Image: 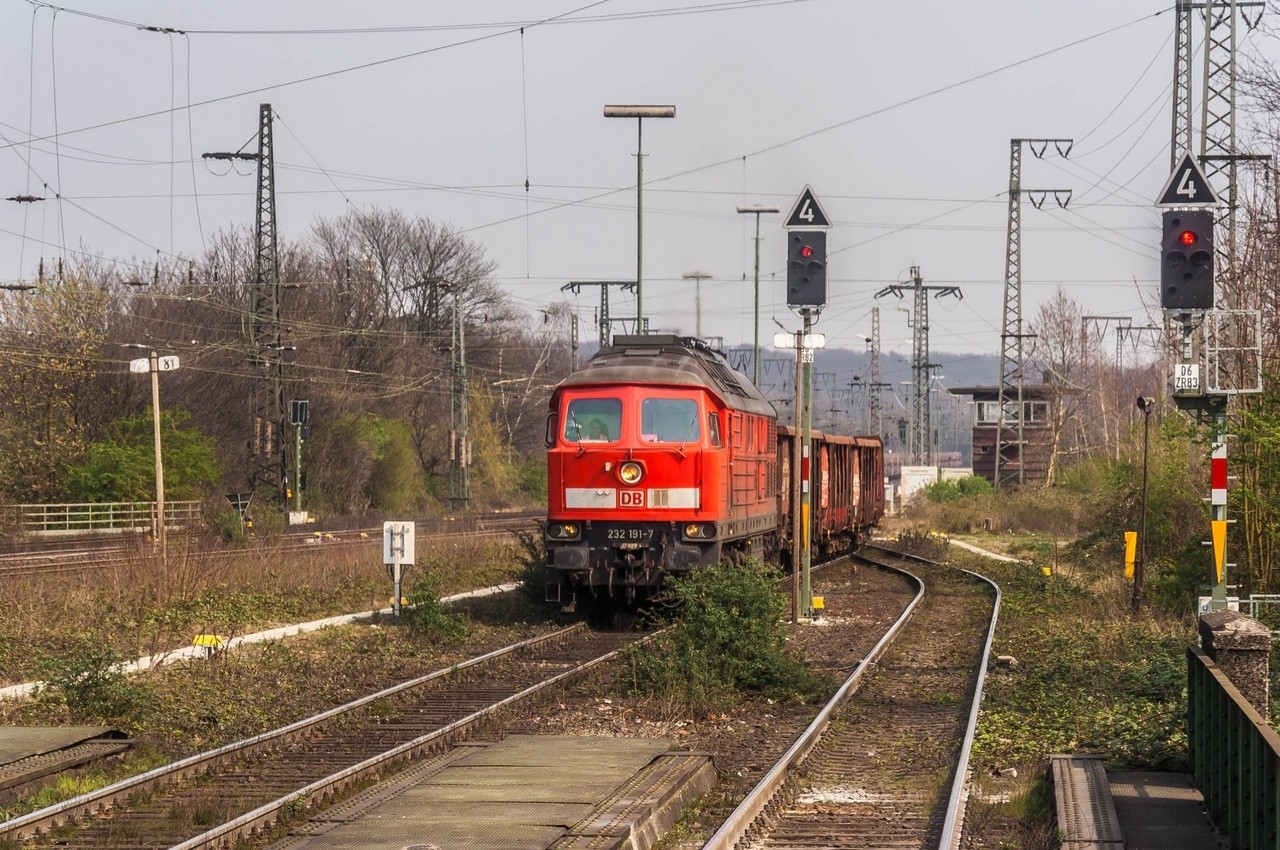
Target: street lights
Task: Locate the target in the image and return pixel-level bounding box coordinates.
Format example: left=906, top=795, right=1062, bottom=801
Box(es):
left=773, top=333, right=826, bottom=624
left=682, top=272, right=713, bottom=337
left=737, top=205, right=780, bottom=389
left=602, top=102, right=677, bottom=335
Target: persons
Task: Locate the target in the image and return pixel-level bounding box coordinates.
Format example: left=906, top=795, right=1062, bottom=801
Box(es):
left=582, top=419, right=608, bottom=440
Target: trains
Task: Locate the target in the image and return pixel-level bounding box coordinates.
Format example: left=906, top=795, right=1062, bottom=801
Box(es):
left=540, top=333, right=886, bottom=621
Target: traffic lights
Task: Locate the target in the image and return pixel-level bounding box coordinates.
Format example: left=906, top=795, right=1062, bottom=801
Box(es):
left=788, top=231, right=827, bottom=305
left=1161, top=211, right=1214, bottom=309
